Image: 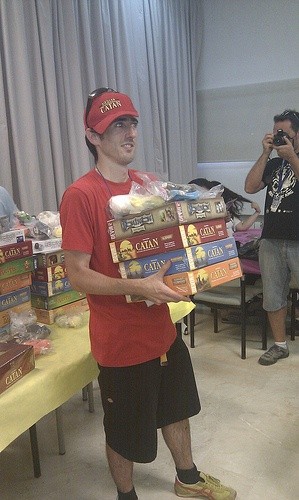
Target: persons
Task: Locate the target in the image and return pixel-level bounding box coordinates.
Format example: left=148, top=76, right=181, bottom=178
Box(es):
left=0, top=186, right=19, bottom=225
left=59, top=88, right=238, bottom=500
left=187, top=178, right=261, bottom=238
left=244, top=109, right=299, bottom=366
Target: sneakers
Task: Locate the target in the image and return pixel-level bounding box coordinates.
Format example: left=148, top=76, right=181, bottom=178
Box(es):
left=258, top=344, right=289, bottom=366
left=174, top=471, right=237, bottom=500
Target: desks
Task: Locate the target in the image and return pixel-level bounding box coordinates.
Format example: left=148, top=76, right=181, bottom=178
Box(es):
left=234, top=230, right=299, bottom=342
left=0, top=301, right=197, bottom=483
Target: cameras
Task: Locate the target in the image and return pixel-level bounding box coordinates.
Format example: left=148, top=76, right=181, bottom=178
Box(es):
left=272, top=130, right=290, bottom=146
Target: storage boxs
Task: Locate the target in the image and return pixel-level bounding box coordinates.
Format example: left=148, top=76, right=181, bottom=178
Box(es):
left=0, top=195, right=244, bottom=396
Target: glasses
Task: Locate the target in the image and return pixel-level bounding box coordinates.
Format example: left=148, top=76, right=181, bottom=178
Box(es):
left=281, top=110, right=299, bottom=123
left=86, top=88, right=119, bottom=128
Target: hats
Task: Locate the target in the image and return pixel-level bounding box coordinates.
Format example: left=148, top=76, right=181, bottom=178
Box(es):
left=84, top=91, right=139, bottom=134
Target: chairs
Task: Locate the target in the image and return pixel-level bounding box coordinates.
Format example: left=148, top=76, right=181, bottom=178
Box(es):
left=188, top=272, right=269, bottom=360
